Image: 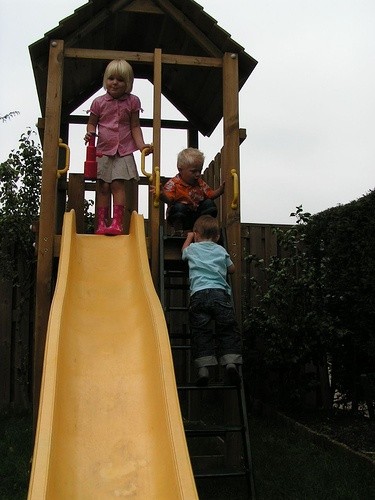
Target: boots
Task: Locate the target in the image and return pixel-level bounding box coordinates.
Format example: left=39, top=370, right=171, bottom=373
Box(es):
left=106, top=204, right=126, bottom=234
left=94, top=207, right=110, bottom=235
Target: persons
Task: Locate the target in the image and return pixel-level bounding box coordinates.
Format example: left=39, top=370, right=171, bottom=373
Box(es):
left=83, top=60, right=153, bottom=235
left=182, top=215, right=243, bottom=387
left=158, top=148, right=226, bottom=236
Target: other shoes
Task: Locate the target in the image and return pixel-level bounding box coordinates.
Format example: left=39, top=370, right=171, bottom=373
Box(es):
left=224, top=364, right=241, bottom=385
left=197, top=368, right=211, bottom=387
